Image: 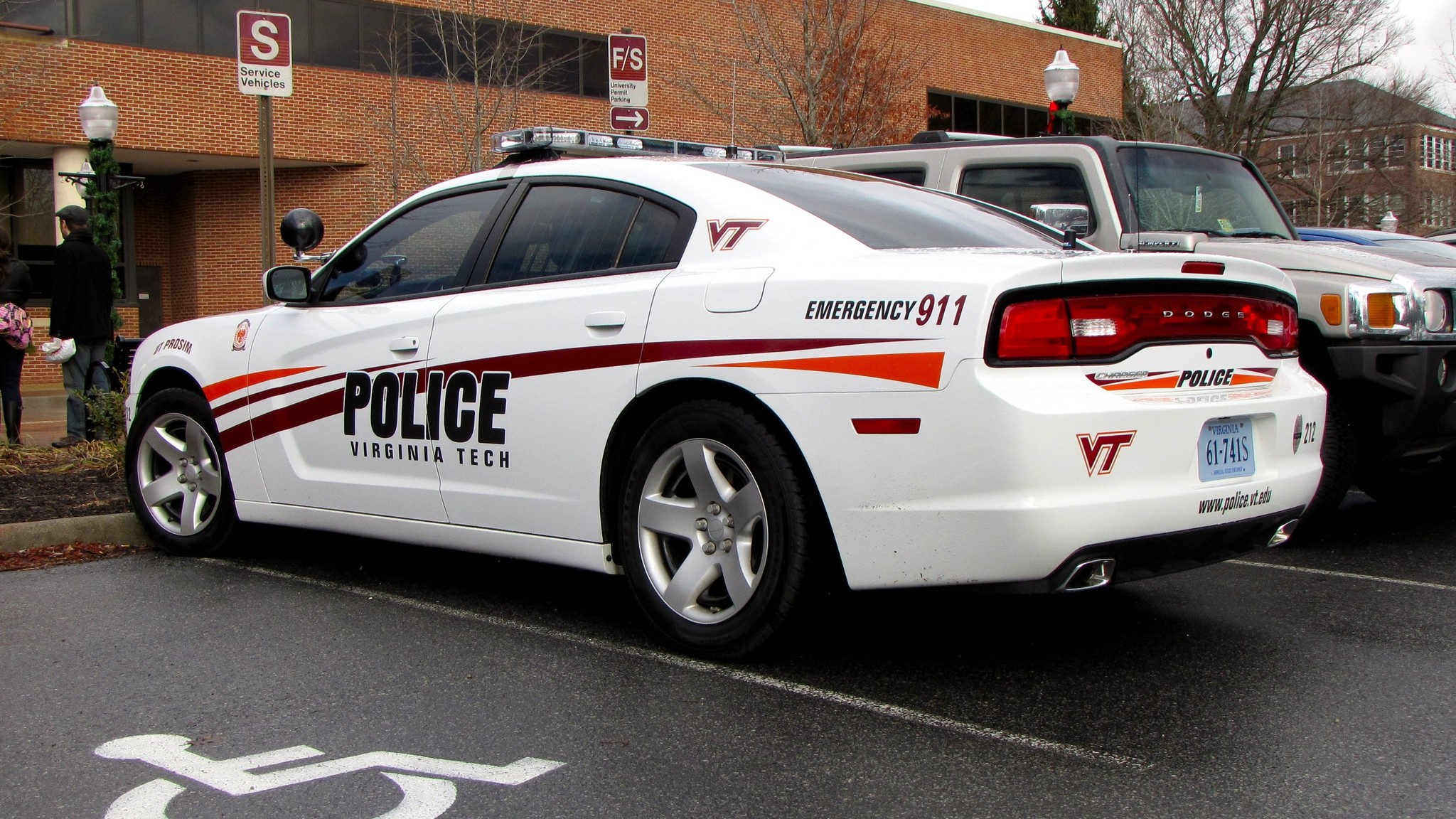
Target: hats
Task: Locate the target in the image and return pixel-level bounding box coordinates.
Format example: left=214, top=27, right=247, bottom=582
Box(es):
left=55, top=205, right=88, bottom=224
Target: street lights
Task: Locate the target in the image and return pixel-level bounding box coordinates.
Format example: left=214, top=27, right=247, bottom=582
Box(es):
left=58, top=86, right=145, bottom=391
left=1044, top=43, right=1080, bottom=137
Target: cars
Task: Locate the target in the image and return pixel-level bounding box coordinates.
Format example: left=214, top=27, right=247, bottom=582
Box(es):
left=1212, top=226, right=1456, bottom=261
left=122, top=126, right=1331, bottom=662
left=1419, top=228, right=1455, bottom=245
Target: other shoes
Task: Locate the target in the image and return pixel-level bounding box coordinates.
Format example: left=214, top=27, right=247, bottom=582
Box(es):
left=10, top=435, right=111, bottom=449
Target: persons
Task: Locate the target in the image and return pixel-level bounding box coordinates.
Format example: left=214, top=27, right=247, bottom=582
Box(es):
left=49, top=205, right=113, bottom=448
left=0, top=227, right=33, bottom=448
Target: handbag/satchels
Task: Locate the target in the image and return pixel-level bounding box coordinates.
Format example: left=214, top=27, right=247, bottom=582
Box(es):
left=0, top=302, right=34, bottom=349
left=42, top=337, right=77, bottom=365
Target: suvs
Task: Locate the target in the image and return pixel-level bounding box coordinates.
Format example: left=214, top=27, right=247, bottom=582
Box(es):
left=773, top=131, right=1456, bottom=549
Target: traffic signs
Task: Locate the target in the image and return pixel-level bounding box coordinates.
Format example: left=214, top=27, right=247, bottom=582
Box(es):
left=608, top=34, right=649, bottom=132
